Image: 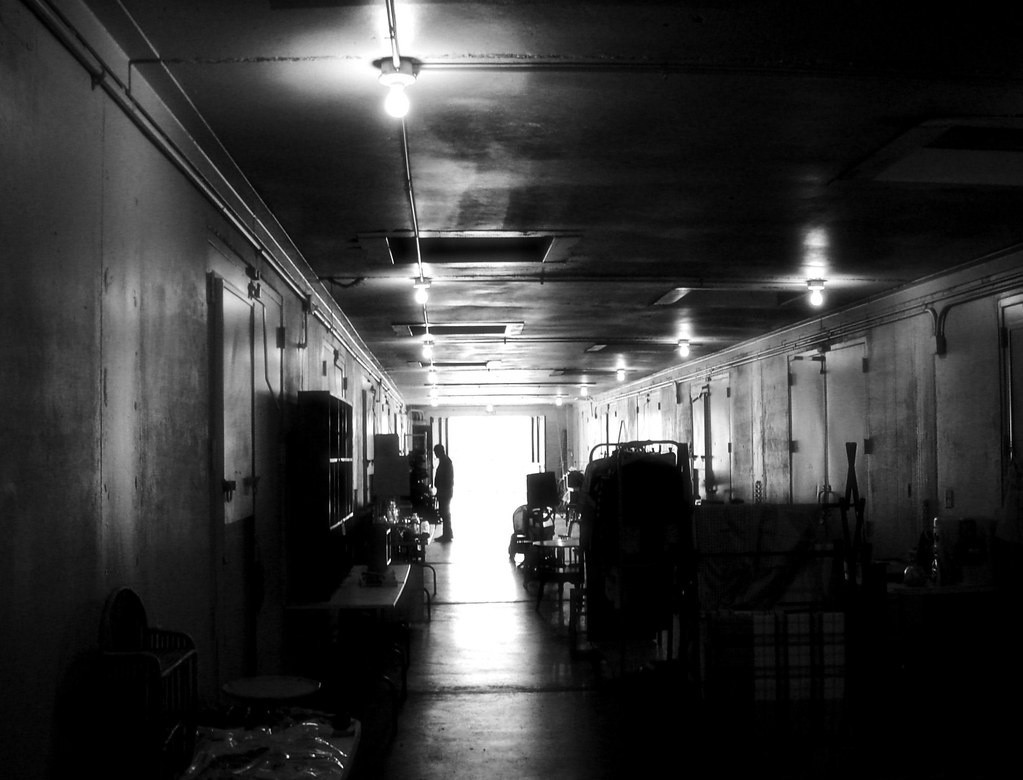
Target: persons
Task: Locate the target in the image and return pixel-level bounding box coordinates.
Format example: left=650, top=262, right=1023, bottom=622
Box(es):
left=433, top=445, right=452, bottom=543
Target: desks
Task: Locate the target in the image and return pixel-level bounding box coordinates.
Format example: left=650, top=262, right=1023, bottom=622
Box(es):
left=325, top=563, right=412, bottom=627
left=181, top=713, right=362, bottom=779
left=533, top=538, right=583, bottom=606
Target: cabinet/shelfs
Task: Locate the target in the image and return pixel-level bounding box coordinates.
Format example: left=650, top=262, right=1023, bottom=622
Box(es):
left=296, top=392, right=355, bottom=529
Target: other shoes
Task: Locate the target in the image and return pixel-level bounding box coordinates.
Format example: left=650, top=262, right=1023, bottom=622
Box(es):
left=435, top=536, right=450, bottom=543
